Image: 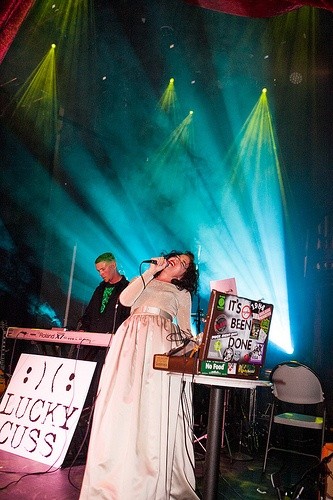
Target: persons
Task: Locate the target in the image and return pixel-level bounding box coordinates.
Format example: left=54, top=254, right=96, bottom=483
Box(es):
left=79, top=250, right=200, bottom=500
left=76, top=252, right=131, bottom=395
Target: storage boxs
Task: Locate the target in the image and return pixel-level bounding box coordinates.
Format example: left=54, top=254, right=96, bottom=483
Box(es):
left=152, top=289, right=274, bottom=380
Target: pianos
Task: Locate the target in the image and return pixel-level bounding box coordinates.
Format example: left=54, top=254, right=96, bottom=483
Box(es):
left=6, top=326, right=116, bottom=456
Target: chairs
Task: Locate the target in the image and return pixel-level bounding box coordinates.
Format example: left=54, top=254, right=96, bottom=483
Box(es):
left=263, top=361, right=328, bottom=490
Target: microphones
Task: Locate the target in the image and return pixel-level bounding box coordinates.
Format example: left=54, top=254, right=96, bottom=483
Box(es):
left=142, top=259, right=170, bottom=268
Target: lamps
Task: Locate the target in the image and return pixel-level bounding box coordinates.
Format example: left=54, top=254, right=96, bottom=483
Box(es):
left=289, top=68, right=304, bottom=84
left=159, top=22, right=179, bottom=38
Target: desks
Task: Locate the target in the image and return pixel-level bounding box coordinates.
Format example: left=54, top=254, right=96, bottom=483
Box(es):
left=168, top=372, right=273, bottom=500
